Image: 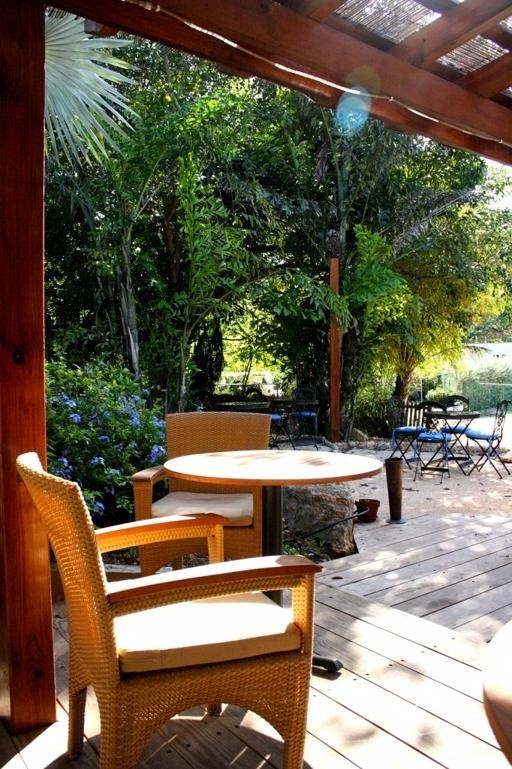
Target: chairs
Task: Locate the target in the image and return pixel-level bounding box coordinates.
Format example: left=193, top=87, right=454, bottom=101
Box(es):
left=129, top=411, right=273, bottom=578
left=387, top=395, right=508, bottom=480
left=15, top=451, right=316, bottom=768
left=243, top=389, right=323, bottom=452
